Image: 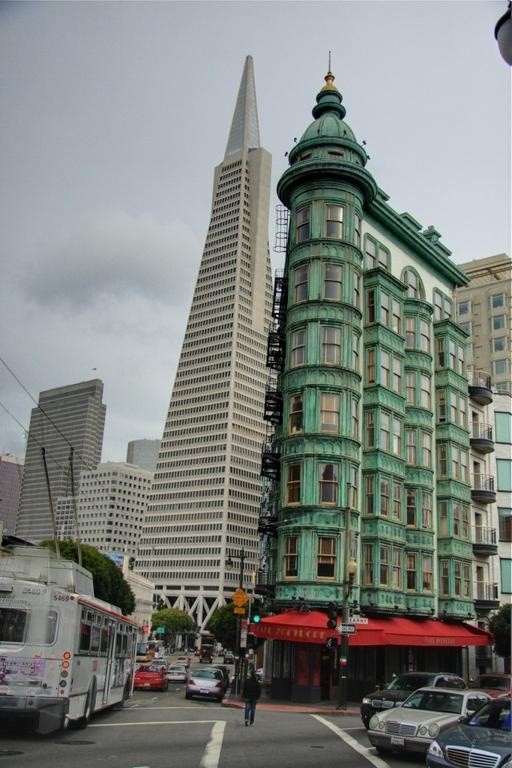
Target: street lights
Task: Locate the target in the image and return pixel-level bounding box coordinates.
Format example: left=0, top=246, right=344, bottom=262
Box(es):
left=337, top=559, right=359, bottom=709
left=225, top=548, right=248, bottom=658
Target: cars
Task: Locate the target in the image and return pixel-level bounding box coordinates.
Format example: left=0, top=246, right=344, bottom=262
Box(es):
left=360, top=673, right=512, bottom=768
left=199, top=644, right=214, bottom=664
left=134, top=654, right=234, bottom=703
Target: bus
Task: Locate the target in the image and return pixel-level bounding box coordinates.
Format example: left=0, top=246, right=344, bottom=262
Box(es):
left=0, top=546, right=140, bottom=736
left=136, top=640, right=165, bottom=662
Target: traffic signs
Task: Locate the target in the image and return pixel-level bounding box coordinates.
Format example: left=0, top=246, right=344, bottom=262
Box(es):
left=337, top=624, right=355, bottom=635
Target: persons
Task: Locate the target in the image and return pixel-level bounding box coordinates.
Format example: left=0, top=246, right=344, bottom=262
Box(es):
left=240, top=669, right=261, bottom=727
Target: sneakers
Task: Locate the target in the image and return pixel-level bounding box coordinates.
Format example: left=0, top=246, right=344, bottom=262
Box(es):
left=244, top=720, right=254, bottom=726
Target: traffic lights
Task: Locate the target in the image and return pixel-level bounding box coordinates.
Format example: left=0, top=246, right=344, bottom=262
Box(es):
left=251, top=598, right=262, bottom=624
left=326, top=600, right=339, bottom=629
left=326, top=637, right=337, bottom=648
